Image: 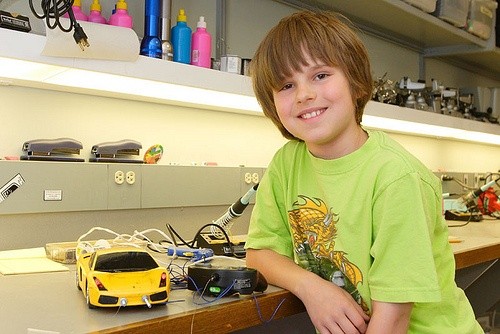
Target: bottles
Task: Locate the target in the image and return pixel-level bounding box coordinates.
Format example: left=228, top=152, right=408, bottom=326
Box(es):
left=88, top=0, right=107, bottom=24
left=171, top=9, right=192, bottom=64
left=64, top=0, right=88, bottom=20
left=192, top=17, right=211, bottom=69
left=109, top=0, right=132, bottom=29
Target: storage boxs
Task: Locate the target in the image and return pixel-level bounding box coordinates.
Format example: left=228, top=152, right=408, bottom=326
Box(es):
left=403, top=0, right=497, bottom=40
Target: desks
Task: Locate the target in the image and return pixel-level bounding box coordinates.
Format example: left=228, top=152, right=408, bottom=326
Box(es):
left=0, top=214, right=500, bottom=334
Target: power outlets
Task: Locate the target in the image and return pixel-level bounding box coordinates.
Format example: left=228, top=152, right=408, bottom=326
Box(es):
left=479, top=175, right=484, bottom=180
left=245, top=173, right=251, bottom=184
left=441, top=175, right=447, bottom=181
left=114, top=171, right=124, bottom=184
left=252, top=173, right=258, bottom=184
left=126, top=171, right=136, bottom=184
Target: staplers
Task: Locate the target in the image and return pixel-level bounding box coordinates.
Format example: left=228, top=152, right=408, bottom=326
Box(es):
left=88, top=137, right=145, bottom=164
left=20, top=136, right=86, bottom=163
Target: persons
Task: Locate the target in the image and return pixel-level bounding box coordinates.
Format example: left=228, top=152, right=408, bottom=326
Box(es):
left=244, top=11, right=486, bottom=334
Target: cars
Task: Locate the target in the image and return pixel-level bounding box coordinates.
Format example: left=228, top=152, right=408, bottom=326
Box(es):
left=76, top=243, right=171, bottom=309
